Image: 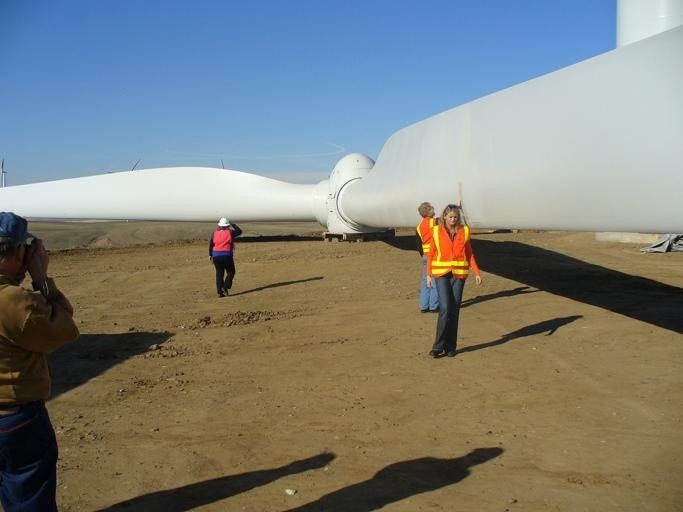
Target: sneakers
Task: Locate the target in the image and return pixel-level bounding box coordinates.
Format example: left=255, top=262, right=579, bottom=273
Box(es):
left=218, top=287, right=228, bottom=297
left=421, top=306, right=439, bottom=313
left=429, top=344, right=457, bottom=357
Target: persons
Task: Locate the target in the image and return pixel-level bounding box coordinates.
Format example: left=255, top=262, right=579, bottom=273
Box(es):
left=415, top=198, right=461, bottom=313
left=208, top=217, right=242, bottom=298
left=0, top=211, right=79, bottom=511
left=426, top=204, right=482, bottom=357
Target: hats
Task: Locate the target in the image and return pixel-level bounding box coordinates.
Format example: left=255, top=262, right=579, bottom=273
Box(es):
left=217, top=217, right=231, bottom=227
left=0, top=212, right=34, bottom=238
